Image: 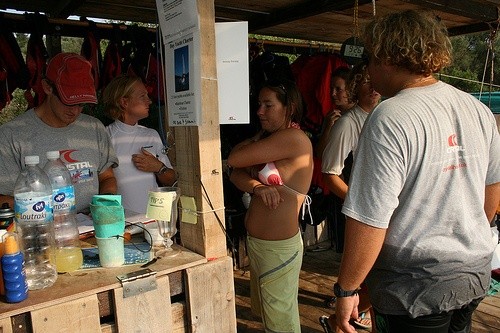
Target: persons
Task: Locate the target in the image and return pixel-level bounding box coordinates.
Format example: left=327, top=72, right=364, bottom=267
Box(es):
left=314, top=8, right=500, bottom=333
left=104, top=74, right=176, bottom=214
left=0, top=52, right=119, bottom=215
left=222, top=80, right=314, bottom=333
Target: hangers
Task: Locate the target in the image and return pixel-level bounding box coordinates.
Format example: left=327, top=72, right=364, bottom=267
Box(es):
left=0, top=11, right=164, bottom=46
left=249, top=38, right=348, bottom=67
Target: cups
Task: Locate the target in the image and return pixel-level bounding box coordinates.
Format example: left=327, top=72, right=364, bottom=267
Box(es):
left=95, top=233, right=124, bottom=269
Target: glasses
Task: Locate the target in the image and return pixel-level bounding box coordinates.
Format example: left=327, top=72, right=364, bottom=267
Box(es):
left=276, top=78, right=288, bottom=93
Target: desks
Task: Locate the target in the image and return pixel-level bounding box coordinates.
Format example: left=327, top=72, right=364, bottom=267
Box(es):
left=0, top=236, right=207, bottom=333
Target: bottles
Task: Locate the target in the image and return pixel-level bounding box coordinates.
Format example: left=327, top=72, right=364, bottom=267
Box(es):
left=43, top=151, right=83, bottom=274
left=13, top=155, right=58, bottom=291
left=1, top=237, right=29, bottom=303
left=0, top=202, right=15, bottom=232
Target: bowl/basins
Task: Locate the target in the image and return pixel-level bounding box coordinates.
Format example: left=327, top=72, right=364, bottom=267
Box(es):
left=143, top=221, right=177, bottom=246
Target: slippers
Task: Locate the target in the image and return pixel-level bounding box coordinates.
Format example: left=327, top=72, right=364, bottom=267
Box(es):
left=354, top=312, right=372, bottom=328
left=319, top=314, right=333, bottom=333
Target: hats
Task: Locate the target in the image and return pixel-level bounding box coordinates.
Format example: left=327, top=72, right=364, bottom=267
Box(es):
left=46, top=52, right=98, bottom=106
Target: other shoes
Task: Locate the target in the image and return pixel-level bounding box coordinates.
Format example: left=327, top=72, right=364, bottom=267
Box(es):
left=327, top=296, right=337, bottom=308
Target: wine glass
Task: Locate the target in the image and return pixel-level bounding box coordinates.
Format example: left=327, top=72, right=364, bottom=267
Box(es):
left=150, top=186, right=181, bottom=260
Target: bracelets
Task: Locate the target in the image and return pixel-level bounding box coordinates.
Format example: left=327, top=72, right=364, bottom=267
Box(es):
left=253, top=184, right=263, bottom=192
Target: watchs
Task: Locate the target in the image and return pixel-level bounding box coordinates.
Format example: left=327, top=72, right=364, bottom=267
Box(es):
left=334, top=283, right=355, bottom=298
left=153, top=163, right=166, bottom=174
